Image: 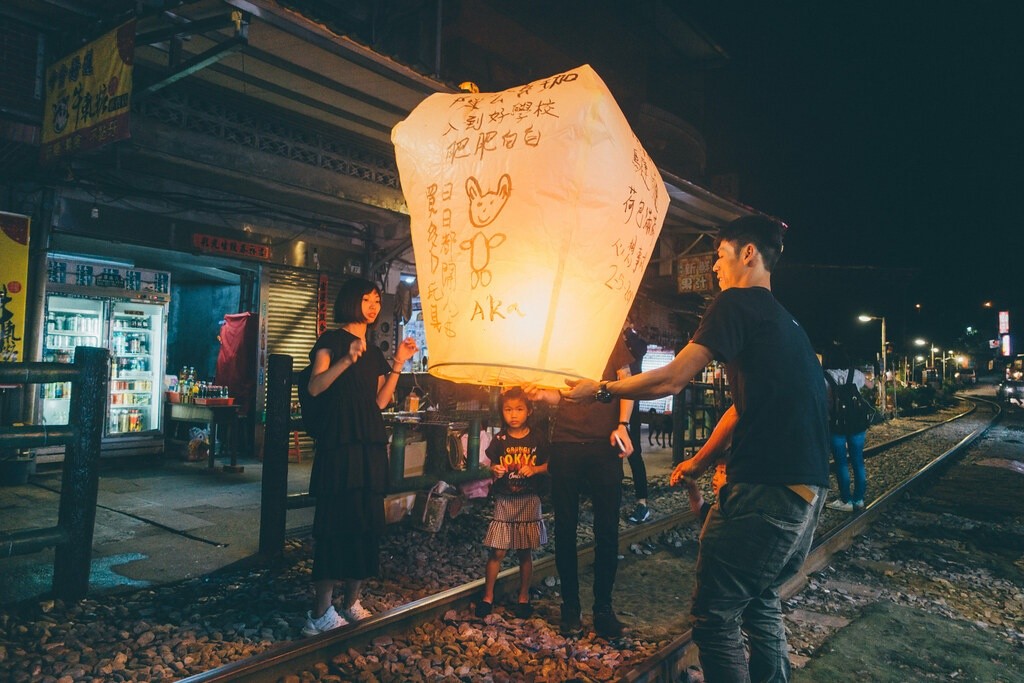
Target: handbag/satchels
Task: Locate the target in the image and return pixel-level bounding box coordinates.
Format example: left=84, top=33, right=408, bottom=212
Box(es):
left=297, top=360, right=327, bottom=446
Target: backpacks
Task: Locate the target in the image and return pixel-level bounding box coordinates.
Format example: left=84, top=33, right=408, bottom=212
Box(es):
left=824, top=368, right=872, bottom=435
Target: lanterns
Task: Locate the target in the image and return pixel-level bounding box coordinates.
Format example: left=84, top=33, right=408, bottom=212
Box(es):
left=392, top=63, right=671, bottom=389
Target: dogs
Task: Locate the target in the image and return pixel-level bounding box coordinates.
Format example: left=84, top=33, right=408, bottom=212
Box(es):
left=648, top=408, right=672, bottom=448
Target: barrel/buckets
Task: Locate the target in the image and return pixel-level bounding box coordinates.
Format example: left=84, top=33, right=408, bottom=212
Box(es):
left=0, top=451, right=37, bottom=486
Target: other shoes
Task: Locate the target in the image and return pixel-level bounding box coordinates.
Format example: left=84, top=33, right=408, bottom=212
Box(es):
left=515, top=599, right=531, bottom=619
left=825, top=500, right=854, bottom=512
left=475, top=600, right=493, bottom=617
left=852, top=500, right=864, bottom=509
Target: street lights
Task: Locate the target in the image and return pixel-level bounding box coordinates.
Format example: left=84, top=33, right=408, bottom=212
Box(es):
left=933, top=347, right=946, bottom=380
left=859, top=314, right=887, bottom=416
left=915, top=338, right=934, bottom=368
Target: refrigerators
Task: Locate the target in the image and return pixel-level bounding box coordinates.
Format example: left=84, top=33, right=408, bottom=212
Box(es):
left=33, top=253, right=172, bottom=443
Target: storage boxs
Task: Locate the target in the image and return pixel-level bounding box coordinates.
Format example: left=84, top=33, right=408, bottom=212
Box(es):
left=383, top=442, right=428, bottom=522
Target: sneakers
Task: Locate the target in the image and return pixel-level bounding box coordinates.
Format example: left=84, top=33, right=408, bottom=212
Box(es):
left=592, top=604, right=623, bottom=640
left=339, top=599, right=372, bottom=623
left=559, top=603, right=585, bottom=635
left=628, top=502, right=649, bottom=522
left=302, top=605, right=350, bottom=637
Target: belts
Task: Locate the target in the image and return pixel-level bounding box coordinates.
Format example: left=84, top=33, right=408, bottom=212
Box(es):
left=785, top=484, right=819, bottom=506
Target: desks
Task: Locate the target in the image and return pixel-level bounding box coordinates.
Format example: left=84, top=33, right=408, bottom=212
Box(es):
left=166, top=402, right=240, bottom=469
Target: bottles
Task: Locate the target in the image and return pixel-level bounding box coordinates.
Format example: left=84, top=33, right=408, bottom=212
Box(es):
left=110, top=409, right=143, bottom=432
left=179, top=365, right=189, bottom=380
left=188, top=367, right=197, bottom=379
left=405, top=392, right=419, bottom=412
left=702, top=365, right=723, bottom=383
left=130, top=316, right=148, bottom=329
left=169, top=378, right=228, bottom=397
left=114, top=332, right=146, bottom=354
left=48, top=312, right=55, bottom=330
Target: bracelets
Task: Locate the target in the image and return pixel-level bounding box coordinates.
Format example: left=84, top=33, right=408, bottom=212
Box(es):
left=393, top=356, right=407, bottom=364
left=391, top=370, right=401, bottom=374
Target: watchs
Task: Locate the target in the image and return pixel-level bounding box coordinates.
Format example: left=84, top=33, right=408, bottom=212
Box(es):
left=618, top=422, right=631, bottom=432
left=594, top=379, right=613, bottom=403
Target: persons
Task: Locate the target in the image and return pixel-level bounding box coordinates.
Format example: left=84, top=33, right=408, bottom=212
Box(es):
left=824, top=344, right=874, bottom=509
left=476, top=386, right=549, bottom=619
left=301, top=278, right=420, bottom=637
left=500, top=215, right=832, bottom=683
